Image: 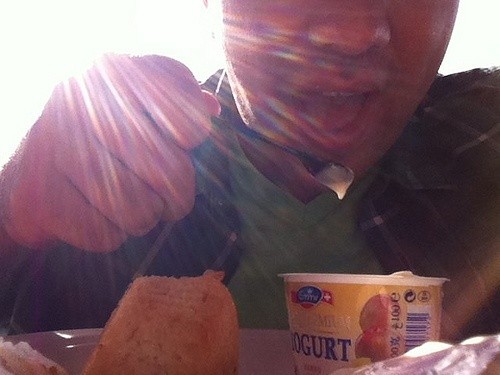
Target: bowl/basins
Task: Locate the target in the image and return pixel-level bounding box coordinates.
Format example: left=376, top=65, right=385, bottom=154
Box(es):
left=278, top=273, right=451, bottom=375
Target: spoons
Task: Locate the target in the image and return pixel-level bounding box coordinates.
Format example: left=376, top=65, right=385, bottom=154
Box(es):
left=209, top=112, right=351, bottom=185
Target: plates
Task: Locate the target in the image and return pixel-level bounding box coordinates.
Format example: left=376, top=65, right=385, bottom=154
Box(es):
left=1, top=328, right=298, bottom=375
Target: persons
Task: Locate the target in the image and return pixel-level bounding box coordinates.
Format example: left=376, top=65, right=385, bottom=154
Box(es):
left=0, top=1, right=499, bottom=374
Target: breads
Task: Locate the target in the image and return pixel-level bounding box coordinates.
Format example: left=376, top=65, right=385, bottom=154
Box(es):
left=79, top=269, right=239, bottom=375
